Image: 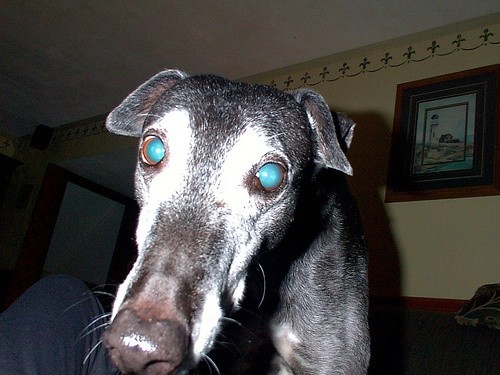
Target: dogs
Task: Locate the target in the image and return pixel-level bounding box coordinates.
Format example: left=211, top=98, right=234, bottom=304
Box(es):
left=102, top=68, right=374, bottom=372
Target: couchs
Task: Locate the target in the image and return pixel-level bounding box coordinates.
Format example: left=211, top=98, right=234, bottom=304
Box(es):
left=369, top=295, right=500, bottom=375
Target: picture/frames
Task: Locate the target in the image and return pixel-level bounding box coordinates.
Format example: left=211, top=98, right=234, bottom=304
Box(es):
left=384, top=63, right=500, bottom=203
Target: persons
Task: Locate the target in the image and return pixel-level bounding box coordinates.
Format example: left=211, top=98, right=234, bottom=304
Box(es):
left=0, top=273, right=123, bottom=375
